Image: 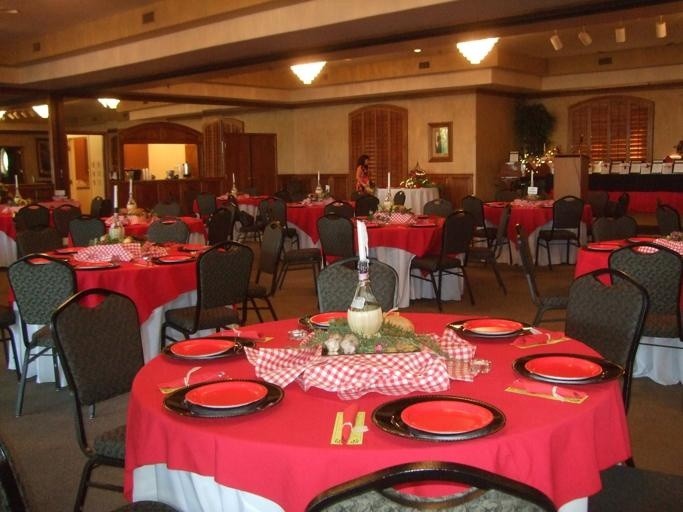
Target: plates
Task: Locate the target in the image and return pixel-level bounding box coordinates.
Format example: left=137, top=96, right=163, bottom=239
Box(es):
left=401, top=401, right=493, bottom=434
left=628, top=237, right=657, bottom=243
left=310, top=313, right=348, bottom=326
left=513, top=354, right=603, bottom=384
left=447, top=318, right=531, bottom=337
left=158, top=255, right=192, bottom=262
left=298, top=313, right=348, bottom=331
left=170, top=379, right=284, bottom=419
left=171, top=339, right=235, bottom=357
left=586, top=244, right=620, bottom=251
left=76, top=261, right=112, bottom=269
left=525, top=356, right=602, bottom=381
left=463, top=319, right=522, bottom=334
left=184, top=381, right=268, bottom=408
left=171, top=335, right=256, bottom=358
left=371, top=394, right=506, bottom=435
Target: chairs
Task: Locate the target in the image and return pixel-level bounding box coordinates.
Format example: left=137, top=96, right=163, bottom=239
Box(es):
left=564, top=267, right=650, bottom=417
left=607, top=241, right=682, bottom=342
left=587, top=465, right=682, bottom=512
left=514, top=222, right=569, bottom=327
left=400, top=186, right=635, bottom=314
left=316, top=186, right=400, bottom=257
left=316, top=257, right=400, bottom=315
left=1, top=185, right=316, bottom=422
left=304, top=461, right=558, bottom=511
left=2, top=440, right=177, bottom=512
left=50, top=287, right=145, bottom=512
left=654, top=197, right=682, bottom=234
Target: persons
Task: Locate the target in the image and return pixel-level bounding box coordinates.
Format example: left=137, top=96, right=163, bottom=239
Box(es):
left=435, top=135, right=447, bottom=153
left=351, top=154, right=370, bottom=195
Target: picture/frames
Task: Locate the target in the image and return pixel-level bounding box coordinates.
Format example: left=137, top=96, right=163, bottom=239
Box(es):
left=428, top=119, right=454, bottom=164
left=36, top=137, right=50, bottom=178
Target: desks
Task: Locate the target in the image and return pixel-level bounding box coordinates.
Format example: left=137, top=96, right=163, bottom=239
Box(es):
left=575, top=235, right=682, bottom=387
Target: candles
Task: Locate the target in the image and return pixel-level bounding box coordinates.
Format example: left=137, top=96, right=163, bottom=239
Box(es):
left=530, top=169, right=534, bottom=187
left=386, top=171, right=393, bottom=189
left=129, top=179, right=133, bottom=193
left=355, top=219, right=370, bottom=261
left=317, top=171, right=321, bottom=186
left=232, top=173, right=236, bottom=183
left=14, top=175, right=19, bottom=188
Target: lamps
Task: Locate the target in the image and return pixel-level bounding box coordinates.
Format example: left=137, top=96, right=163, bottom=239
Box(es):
left=289, top=60, right=327, bottom=86
left=455, top=38, right=500, bottom=65
left=547, top=12, right=669, bottom=51
left=31, top=104, right=48, bottom=120
left=98, top=99, right=120, bottom=110
